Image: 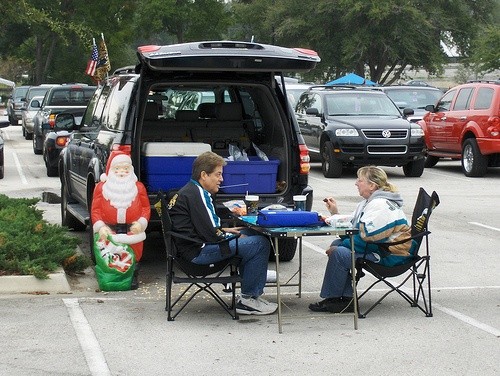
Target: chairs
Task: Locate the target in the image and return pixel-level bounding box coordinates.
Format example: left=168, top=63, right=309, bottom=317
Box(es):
left=143, top=103, right=159, bottom=122
left=340, top=187, right=441, bottom=320
left=53, top=94, right=67, bottom=105
left=157, top=193, right=240, bottom=321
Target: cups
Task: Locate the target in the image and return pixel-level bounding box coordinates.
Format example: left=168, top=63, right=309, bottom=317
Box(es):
left=293, top=195, right=307, bottom=211
left=245, top=196, right=259, bottom=216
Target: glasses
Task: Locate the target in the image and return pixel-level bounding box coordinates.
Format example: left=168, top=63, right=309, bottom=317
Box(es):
left=357, top=178, right=369, bottom=183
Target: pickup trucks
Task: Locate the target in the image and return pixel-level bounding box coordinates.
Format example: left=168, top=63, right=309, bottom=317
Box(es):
left=31, top=84, right=98, bottom=162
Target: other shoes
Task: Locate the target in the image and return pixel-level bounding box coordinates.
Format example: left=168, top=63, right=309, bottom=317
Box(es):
left=309, top=298, right=341, bottom=311
left=327, top=297, right=354, bottom=312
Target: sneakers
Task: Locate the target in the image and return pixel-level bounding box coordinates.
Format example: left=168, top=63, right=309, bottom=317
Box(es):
left=235, top=294, right=278, bottom=314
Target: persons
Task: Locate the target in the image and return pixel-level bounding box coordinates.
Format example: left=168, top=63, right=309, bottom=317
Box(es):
left=309, top=166, right=412, bottom=313
left=167, top=151, right=279, bottom=315
left=92, top=151, right=150, bottom=289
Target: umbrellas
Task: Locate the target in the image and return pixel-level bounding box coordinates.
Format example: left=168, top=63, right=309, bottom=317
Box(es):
left=326, top=73, right=376, bottom=85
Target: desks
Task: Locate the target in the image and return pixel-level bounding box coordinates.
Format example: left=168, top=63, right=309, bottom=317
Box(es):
left=233, top=211, right=360, bottom=334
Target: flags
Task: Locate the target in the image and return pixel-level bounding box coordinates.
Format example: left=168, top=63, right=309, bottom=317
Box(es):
left=86, top=41, right=111, bottom=79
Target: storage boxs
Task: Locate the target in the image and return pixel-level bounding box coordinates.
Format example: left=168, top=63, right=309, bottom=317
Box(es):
left=142, top=140, right=212, bottom=193
left=222, top=152, right=281, bottom=194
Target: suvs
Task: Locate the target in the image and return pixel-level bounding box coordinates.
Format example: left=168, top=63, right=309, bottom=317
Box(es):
left=357, top=85, right=444, bottom=122
left=416, top=79, right=500, bottom=176
left=55, top=37, right=321, bottom=265
left=278, top=79, right=428, bottom=178
left=7, top=84, right=62, bottom=125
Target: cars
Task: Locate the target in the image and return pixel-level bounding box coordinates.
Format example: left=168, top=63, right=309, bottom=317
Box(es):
left=42, top=116, right=84, bottom=177
left=20, top=96, right=45, bottom=140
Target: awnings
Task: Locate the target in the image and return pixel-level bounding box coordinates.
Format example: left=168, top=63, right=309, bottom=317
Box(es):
left=0, top=78, right=15, bottom=88
